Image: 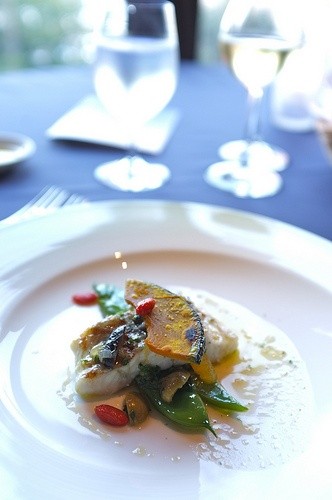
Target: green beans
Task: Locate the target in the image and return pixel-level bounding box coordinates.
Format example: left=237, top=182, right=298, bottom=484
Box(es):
left=137, top=369, right=248, bottom=439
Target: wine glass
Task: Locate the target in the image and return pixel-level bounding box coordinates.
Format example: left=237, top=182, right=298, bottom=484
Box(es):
left=94, top=0, right=180, bottom=191
left=205, top=0, right=300, bottom=197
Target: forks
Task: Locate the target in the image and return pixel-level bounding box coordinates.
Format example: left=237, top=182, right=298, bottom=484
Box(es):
left=25, top=184, right=90, bottom=208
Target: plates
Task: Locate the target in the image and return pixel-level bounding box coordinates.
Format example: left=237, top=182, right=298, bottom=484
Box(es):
left=0, top=199, right=332, bottom=499
left=47, top=93, right=180, bottom=153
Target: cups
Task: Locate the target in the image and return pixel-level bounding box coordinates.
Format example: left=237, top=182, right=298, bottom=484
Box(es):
left=314, top=85, right=332, bottom=162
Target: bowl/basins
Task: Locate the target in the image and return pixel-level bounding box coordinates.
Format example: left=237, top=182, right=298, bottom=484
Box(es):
left=0, top=132, right=37, bottom=173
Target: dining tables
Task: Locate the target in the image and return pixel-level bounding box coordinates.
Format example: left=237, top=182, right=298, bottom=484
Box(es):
left=0, top=60, right=332, bottom=241
